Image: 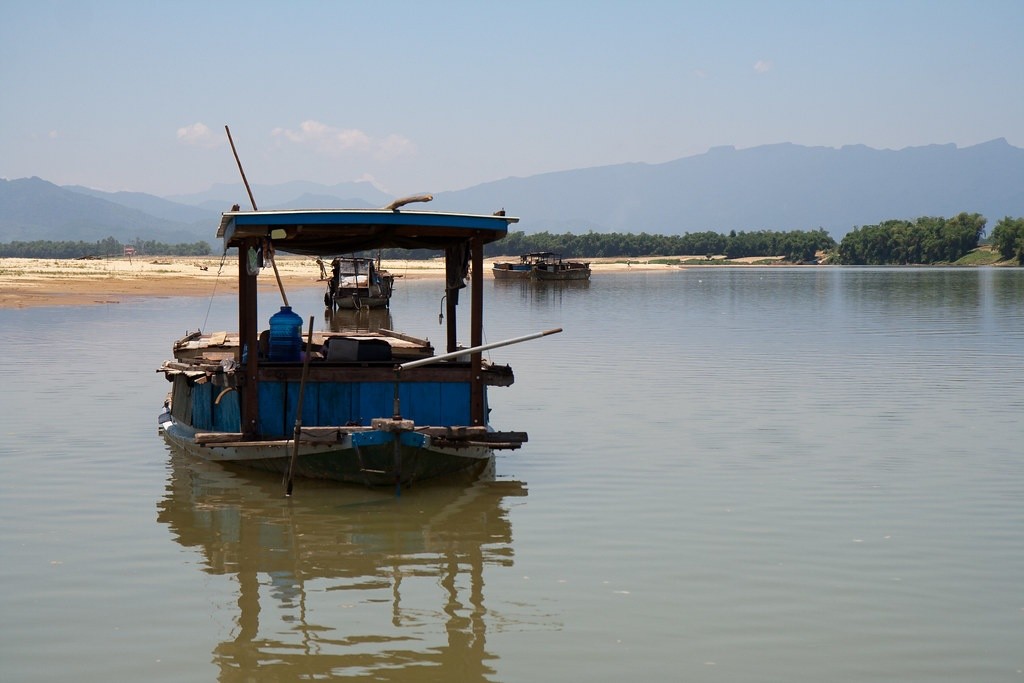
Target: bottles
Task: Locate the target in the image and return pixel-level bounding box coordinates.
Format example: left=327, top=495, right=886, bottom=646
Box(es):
left=268, top=306, right=303, bottom=361
left=220, top=359, right=241, bottom=372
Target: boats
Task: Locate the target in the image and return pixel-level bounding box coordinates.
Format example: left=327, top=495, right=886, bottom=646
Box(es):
left=531, top=252, right=592, bottom=280
left=492, top=251, right=535, bottom=279
left=311, top=249, right=404, bottom=309
left=155, top=195, right=563, bottom=497
